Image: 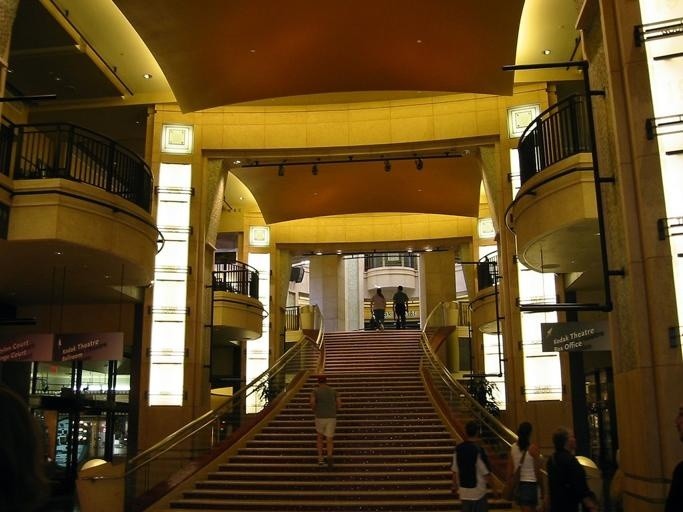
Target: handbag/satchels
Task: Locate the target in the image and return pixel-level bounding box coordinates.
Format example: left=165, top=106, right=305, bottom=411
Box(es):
left=370, top=318, right=377, bottom=329
left=502, top=468, right=521, bottom=500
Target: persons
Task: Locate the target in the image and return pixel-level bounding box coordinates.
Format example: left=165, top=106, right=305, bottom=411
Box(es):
left=501, top=420, right=545, bottom=511
left=661, top=405, right=682, bottom=512
left=371, top=288, right=385, bottom=331
left=311, top=375, right=342, bottom=465
left=450, top=419, right=499, bottom=511
left=392, top=285, right=409, bottom=329
left=545, top=427, right=594, bottom=511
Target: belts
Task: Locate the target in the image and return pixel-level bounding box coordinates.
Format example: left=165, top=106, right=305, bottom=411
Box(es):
left=519, top=481, right=537, bottom=486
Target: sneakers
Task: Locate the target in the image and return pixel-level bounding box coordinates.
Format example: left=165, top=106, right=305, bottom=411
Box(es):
left=318, top=456, right=333, bottom=467
